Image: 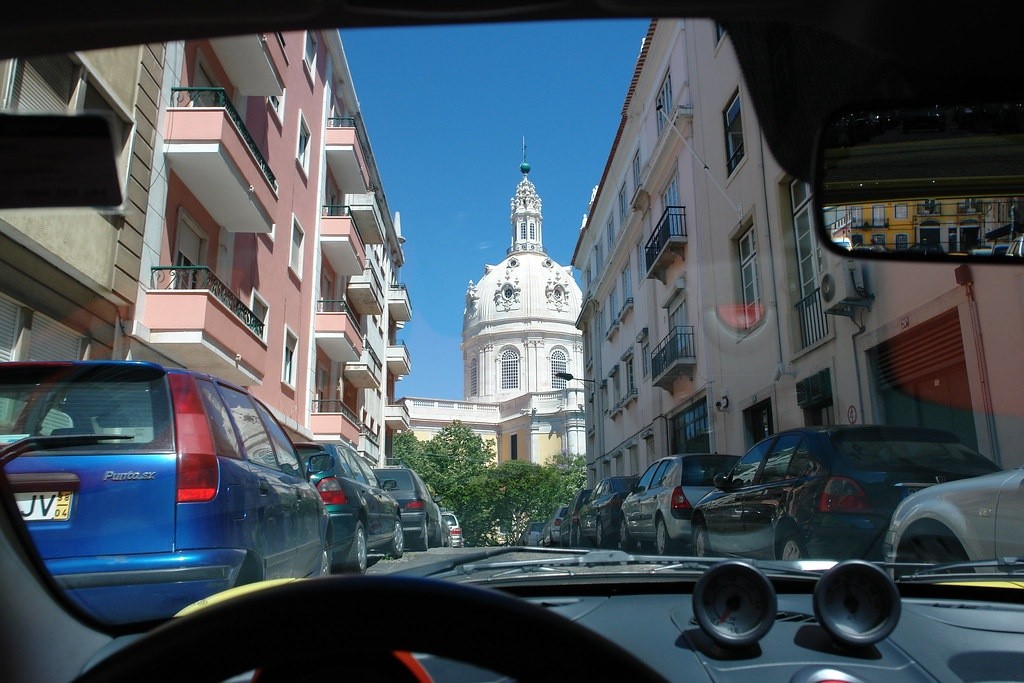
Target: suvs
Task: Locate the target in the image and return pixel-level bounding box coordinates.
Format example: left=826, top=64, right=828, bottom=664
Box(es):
left=292, top=441, right=403, bottom=575
left=559, top=488, right=596, bottom=543
left=0, top=362, right=333, bottom=628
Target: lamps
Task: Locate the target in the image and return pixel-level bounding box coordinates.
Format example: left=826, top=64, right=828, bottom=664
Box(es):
left=554, top=373, right=595, bottom=384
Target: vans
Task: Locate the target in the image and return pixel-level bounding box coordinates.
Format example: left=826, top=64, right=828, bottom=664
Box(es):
left=540, top=506, right=568, bottom=546
left=440, top=514, right=463, bottom=548
left=619, top=453, right=739, bottom=556
left=373, top=469, right=443, bottom=551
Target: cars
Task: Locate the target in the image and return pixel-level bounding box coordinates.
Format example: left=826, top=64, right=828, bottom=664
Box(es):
left=692, top=423, right=1005, bottom=562
left=883, top=466, right=1024, bottom=577
left=519, top=523, right=545, bottom=546
left=577, top=475, right=644, bottom=548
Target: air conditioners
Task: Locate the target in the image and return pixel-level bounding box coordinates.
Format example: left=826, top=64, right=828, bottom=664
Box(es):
left=818, top=261, right=864, bottom=313
